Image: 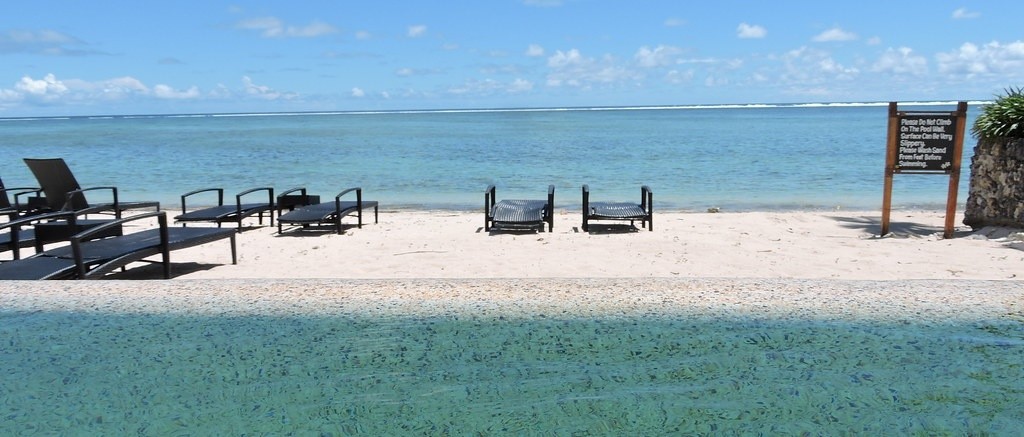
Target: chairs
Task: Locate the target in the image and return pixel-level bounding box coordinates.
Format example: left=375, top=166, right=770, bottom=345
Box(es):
left=0, top=210, right=122, bottom=254
left=174, top=186, right=278, bottom=233
left=22, top=186, right=160, bottom=236
left=581, top=184, right=653, bottom=232
left=24, top=158, right=160, bottom=223
left=276, top=187, right=379, bottom=236
left=0, top=178, right=43, bottom=208
left=0, top=212, right=237, bottom=280
left=484, top=185, right=555, bottom=233
